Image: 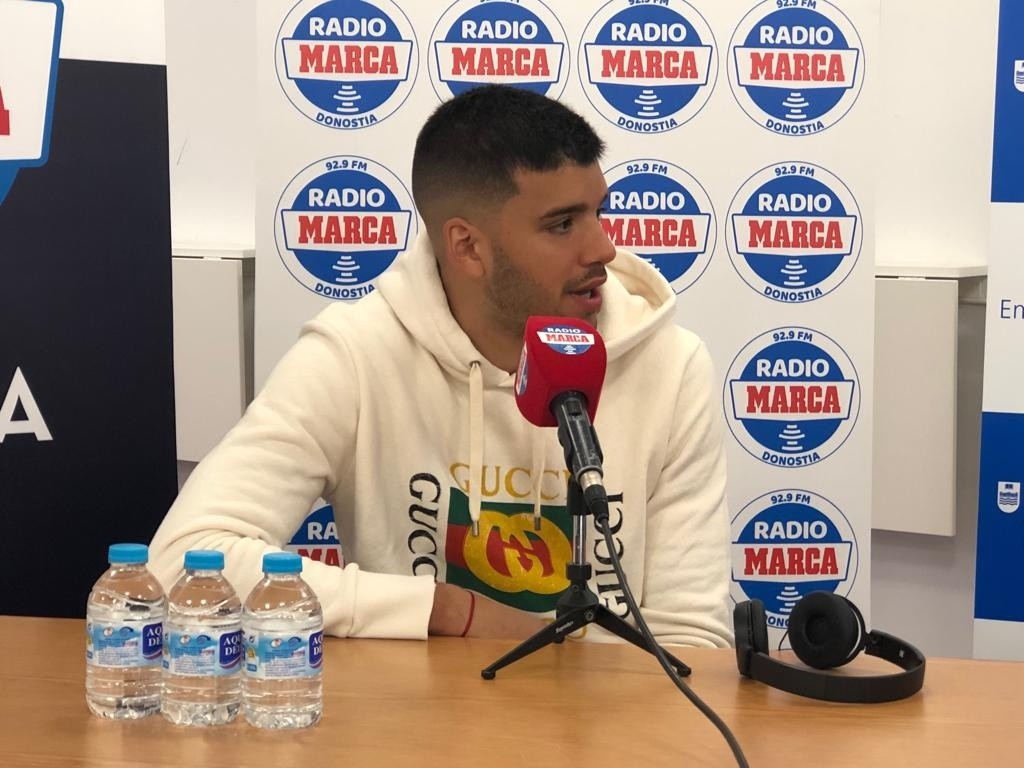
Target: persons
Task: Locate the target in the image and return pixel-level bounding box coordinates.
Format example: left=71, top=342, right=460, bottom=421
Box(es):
left=144, top=82, right=734, bottom=648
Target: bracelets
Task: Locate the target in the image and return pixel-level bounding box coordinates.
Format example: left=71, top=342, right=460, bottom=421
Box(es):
left=460, top=589, right=475, bottom=636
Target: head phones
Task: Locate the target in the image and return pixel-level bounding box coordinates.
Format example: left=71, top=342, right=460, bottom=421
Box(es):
left=734, top=590, right=927, bottom=704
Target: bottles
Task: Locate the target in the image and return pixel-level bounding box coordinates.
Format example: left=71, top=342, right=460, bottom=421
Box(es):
left=158, top=549, right=242, bottom=728
left=83, top=543, right=169, bottom=720
left=239, top=552, right=325, bottom=729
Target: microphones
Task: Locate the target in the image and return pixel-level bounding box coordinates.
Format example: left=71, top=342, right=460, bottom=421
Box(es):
left=512, top=315, right=611, bottom=522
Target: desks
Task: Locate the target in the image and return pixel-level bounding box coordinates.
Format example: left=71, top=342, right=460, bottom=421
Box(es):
left=0, top=613, right=1024, bottom=768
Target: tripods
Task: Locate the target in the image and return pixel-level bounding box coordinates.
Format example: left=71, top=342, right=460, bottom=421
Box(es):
left=478, top=427, right=691, bottom=680
